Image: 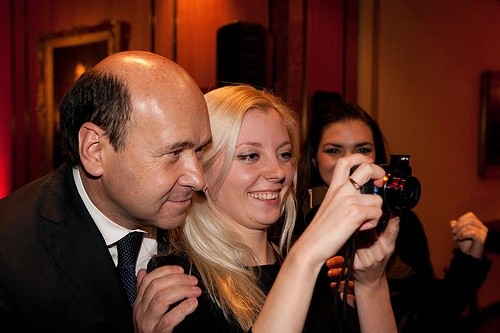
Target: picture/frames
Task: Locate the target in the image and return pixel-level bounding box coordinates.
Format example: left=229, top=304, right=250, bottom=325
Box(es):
left=33, top=19, right=128, bottom=176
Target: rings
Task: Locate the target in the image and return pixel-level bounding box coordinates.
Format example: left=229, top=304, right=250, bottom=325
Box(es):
left=348, top=176, right=360, bottom=190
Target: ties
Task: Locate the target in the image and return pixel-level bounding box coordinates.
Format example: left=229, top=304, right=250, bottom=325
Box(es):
left=116, top=231, right=145, bottom=307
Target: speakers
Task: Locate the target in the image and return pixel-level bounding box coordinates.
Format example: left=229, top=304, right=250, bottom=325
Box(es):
left=214, top=23, right=265, bottom=90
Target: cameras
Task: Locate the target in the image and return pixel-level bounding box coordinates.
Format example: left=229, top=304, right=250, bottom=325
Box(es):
left=351, top=154, right=421, bottom=212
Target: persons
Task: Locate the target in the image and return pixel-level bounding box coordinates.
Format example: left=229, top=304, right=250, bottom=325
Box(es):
left=299, top=102, right=493, bottom=333
left=1, top=51, right=356, bottom=333
left=146, top=85, right=402, bottom=333
